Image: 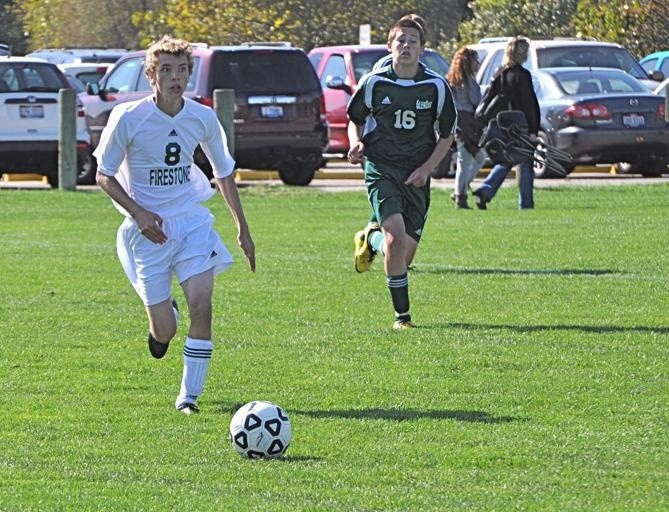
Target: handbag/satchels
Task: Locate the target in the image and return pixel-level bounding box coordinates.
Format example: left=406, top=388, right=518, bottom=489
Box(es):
left=473, top=87, right=510, bottom=127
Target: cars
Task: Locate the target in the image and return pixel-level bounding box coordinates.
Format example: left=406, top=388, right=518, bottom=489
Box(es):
left=306, top=42, right=454, bottom=169
left=0, top=54, right=88, bottom=190
left=628, top=50, right=668, bottom=78
left=616, top=76, right=668, bottom=172
left=0, top=42, right=143, bottom=108
left=528, top=65, right=668, bottom=178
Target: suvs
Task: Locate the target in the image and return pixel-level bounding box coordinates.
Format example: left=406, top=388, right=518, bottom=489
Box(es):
left=76, top=38, right=329, bottom=186
left=450, top=32, right=666, bottom=105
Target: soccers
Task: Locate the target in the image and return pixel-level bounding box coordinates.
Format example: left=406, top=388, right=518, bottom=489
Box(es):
left=228, top=400, right=294, bottom=459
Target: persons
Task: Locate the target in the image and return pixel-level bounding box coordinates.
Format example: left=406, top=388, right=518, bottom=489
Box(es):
left=445, top=49, right=487, bottom=210
left=374, top=15, right=449, bottom=76
left=345, top=21, right=458, bottom=332
left=474, top=39, right=541, bottom=208
left=92, top=36, right=256, bottom=416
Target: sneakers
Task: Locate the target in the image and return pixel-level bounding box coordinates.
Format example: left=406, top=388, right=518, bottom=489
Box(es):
left=449, top=192, right=473, bottom=209
left=472, top=188, right=487, bottom=209
left=354, top=222, right=380, bottom=273
left=393, top=319, right=414, bottom=329
left=149, top=299, right=178, bottom=359
